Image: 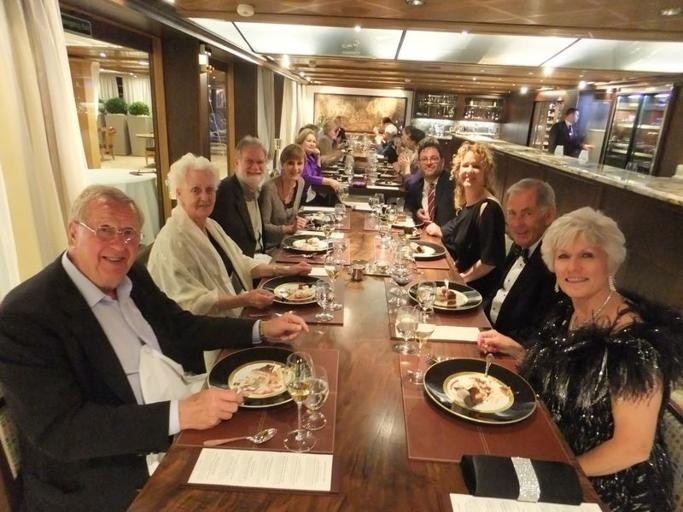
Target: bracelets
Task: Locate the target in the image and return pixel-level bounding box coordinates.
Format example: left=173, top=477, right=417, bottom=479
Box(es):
left=272, top=264, right=279, bottom=276
left=258, top=322, right=267, bottom=342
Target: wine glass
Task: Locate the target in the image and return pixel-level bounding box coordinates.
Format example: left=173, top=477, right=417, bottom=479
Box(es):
left=344, top=156, right=356, bottom=184
left=284, top=352, right=318, bottom=452
left=413, top=307, right=436, bottom=359
left=302, top=366, right=329, bottom=430
left=376, top=227, right=410, bottom=304
left=368, top=153, right=377, bottom=184
left=393, top=306, right=418, bottom=355
left=416, top=281, right=435, bottom=332
left=324, top=255, right=342, bottom=311
left=315, top=280, right=334, bottom=321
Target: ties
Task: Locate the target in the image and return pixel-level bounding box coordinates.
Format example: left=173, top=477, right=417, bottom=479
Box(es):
left=568, top=125, right=571, bottom=134
left=428, top=183, right=436, bottom=221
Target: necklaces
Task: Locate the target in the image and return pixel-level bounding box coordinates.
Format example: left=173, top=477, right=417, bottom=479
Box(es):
left=278, top=176, right=292, bottom=203
left=569, top=287, right=618, bottom=331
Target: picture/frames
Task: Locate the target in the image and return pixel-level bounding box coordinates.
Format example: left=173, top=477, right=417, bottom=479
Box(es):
left=311, top=92, right=409, bottom=136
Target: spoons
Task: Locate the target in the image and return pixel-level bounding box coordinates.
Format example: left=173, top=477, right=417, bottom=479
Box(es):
left=203, top=428, right=277, bottom=447
left=285, top=252, right=317, bottom=259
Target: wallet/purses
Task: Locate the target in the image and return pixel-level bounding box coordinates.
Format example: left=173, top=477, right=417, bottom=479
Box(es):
left=460, top=454, right=582, bottom=505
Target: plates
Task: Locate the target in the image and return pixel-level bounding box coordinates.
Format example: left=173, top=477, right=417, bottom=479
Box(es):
left=411, top=240, right=446, bottom=259
left=261, top=275, right=330, bottom=305
left=392, top=217, right=424, bottom=227
left=282, top=235, right=334, bottom=252
left=408, top=280, right=483, bottom=311
left=207, top=347, right=311, bottom=409
left=424, top=359, right=537, bottom=426
left=297, top=211, right=343, bottom=222
left=376, top=180, right=403, bottom=186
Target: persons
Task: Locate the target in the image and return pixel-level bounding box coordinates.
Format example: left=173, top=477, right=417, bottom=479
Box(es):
left=446, top=143, right=505, bottom=307
left=547, top=108, right=594, bottom=159
left=216, top=133, right=270, bottom=261
left=0, top=184, right=311, bottom=512
left=475, top=204, right=682, bottom=511
left=145, top=152, right=311, bottom=373
left=292, top=113, right=424, bottom=205
left=406, top=138, right=457, bottom=259
left=259, top=139, right=308, bottom=253
left=481, top=179, right=558, bottom=338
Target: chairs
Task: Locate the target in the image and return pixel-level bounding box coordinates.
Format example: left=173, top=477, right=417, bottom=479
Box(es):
left=1, top=396, right=24, bottom=511
left=655, top=393, right=683, bottom=512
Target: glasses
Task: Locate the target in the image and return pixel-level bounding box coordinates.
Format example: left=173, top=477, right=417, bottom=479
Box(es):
left=80, top=223, right=141, bottom=242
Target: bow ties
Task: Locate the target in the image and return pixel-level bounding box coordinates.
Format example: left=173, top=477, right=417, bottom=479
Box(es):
left=512, top=245, right=529, bottom=258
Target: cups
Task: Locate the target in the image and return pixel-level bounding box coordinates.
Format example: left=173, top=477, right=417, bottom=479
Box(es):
left=347, top=260, right=370, bottom=282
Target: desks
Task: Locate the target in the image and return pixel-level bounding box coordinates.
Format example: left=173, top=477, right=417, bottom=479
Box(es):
left=85, top=125, right=158, bottom=246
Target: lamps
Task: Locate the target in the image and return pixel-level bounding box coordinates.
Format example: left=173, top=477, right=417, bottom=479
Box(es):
left=197, top=43, right=209, bottom=73
